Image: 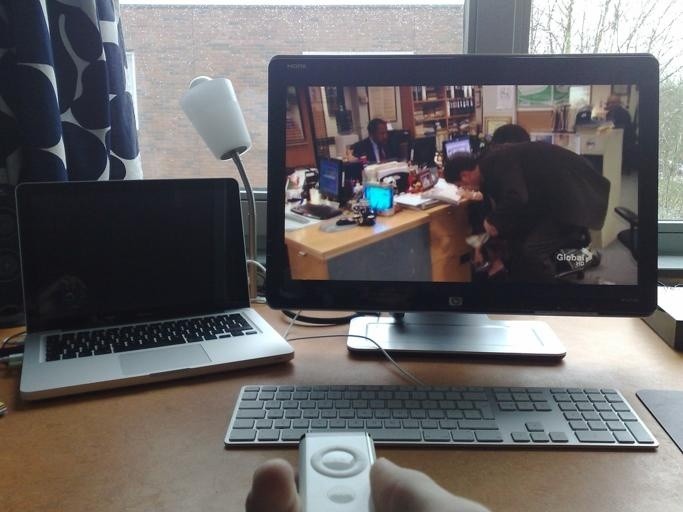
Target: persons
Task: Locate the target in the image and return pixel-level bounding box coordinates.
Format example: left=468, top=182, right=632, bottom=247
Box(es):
left=347, top=116, right=396, bottom=165
left=441, top=142, right=611, bottom=279
left=602, top=93, right=635, bottom=145
left=473, top=122, right=538, bottom=279
left=240, top=451, right=494, bottom=512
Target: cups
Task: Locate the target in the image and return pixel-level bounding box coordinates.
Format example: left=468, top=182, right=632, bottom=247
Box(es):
left=359, top=155, right=369, bottom=167
left=352, top=198, right=379, bottom=225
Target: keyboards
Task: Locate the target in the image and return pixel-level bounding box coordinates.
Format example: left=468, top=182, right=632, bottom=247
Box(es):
left=224, top=381, right=661, bottom=454
left=291, top=203, right=342, bottom=220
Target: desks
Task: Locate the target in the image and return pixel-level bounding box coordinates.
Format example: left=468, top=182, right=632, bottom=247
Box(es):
left=0, top=296, right=682, bottom=512
left=285, top=197, right=471, bottom=281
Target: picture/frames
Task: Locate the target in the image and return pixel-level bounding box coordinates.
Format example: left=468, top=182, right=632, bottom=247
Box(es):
left=418, top=170, right=434, bottom=190
left=484, top=116, right=512, bottom=136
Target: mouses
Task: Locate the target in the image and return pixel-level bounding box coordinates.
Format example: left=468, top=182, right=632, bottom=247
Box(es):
left=336, top=217, right=356, bottom=226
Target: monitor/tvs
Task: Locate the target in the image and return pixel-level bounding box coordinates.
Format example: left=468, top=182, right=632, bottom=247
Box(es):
left=317, top=156, right=343, bottom=203
left=409, top=136, right=436, bottom=169
left=442, top=135, right=472, bottom=159
left=263, top=51, right=659, bottom=364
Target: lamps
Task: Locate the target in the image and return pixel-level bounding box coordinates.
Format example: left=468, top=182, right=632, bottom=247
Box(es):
left=179, top=73, right=264, bottom=298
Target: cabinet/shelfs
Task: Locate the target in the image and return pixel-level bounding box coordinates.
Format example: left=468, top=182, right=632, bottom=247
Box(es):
left=400, top=86, right=483, bottom=139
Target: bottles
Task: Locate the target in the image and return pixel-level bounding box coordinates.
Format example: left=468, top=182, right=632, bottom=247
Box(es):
left=407, top=168, right=419, bottom=186
left=434, top=121, right=441, bottom=131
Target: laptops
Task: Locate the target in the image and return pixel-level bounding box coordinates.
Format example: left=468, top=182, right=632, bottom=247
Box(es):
left=10, top=177, right=294, bottom=402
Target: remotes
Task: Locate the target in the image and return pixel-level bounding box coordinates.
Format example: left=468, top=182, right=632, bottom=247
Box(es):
left=297, top=427, right=381, bottom=512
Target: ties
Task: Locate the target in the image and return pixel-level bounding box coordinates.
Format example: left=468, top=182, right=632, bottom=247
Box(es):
left=377, top=144, right=384, bottom=161
